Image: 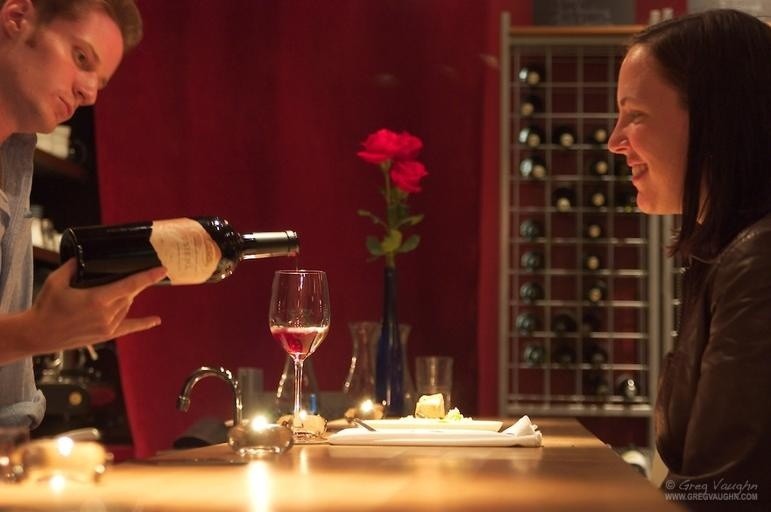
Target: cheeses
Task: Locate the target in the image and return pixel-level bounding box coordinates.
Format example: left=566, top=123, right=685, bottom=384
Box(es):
left=415, top=394, right=445, bottom=419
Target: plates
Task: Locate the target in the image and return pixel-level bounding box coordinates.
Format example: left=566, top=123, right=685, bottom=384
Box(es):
left=349, top=417, right=504, bottom=433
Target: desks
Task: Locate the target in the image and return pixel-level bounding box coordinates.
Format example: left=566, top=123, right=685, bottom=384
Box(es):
left=1, top=417, right=687, bottom=510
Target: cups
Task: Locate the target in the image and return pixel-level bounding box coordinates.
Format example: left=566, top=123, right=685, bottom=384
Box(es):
left=0, top=427, right=30, bottom=485
left=414, top=353, right=455, bottom=416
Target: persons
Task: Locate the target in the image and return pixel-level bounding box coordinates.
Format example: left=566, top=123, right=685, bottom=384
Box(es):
left=605, top=10, right=771, bottom=512
left=1, top=0, right=172, bottom=450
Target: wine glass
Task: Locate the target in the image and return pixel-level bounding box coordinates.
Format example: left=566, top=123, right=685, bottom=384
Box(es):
left=269, top=266, right=334, bottom=442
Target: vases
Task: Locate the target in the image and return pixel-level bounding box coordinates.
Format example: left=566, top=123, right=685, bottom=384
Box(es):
left=375, top=268, right=404, bottom=418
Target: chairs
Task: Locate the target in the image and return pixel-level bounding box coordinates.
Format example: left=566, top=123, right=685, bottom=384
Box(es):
left=35, top=385, right=91, bottom=436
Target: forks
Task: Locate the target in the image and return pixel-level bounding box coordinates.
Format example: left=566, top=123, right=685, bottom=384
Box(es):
left=348, top=415, right=376, bottom=431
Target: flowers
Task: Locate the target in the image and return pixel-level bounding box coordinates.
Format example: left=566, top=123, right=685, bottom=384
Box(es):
left=355, top=128, right=431, bottom=268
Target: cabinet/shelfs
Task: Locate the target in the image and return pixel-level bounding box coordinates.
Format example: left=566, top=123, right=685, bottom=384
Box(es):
left=31, top=147, right=119, bottom=407
left=477, top=8, right=687, bottom=417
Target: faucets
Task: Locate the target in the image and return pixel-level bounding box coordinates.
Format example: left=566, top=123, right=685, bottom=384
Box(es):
left=176, top=364, right=242, bottom=426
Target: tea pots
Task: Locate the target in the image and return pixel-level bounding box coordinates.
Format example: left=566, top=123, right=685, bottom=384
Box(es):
left=347, top=319, right=416, bottom=410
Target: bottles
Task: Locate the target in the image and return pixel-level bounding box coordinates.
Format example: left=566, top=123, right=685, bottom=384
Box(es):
left=517, top=61, right=545, bottom=87
left=552, top=187, right=577, bottom=212
left=518, top=279, right=544, bottom=305
left=514, top=310, right=541, bottom=338
left=583, top=253, right=603, bottom=273
left=614, top=372, right=644, bottom=399
left=585, top=122, right=610, bottom=146
left=583, top=156, right=608, bottom=174
left=520, top=343, right=546, bottom=367
left=514, top=124, right=545, bottom=149
left=517, top=156, right=549, bottom=182
left=513, top=92, right=545, bottom=120
left=580, top=188, right=606, bottom=210
left=552, top=123, right=578, bottom=149
left=57, top=213, right=303, bottom=288
left=582, top=217, right=607, bottom=240
left=583, top=281, right=609, bottom=303
left=518, top=250, right=545, bottom=275
left=518, top=218, right=544, bottom=243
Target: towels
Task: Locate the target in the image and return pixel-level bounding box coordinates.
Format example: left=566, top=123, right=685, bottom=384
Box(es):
left=327, top=414, right=543, bottom=447
